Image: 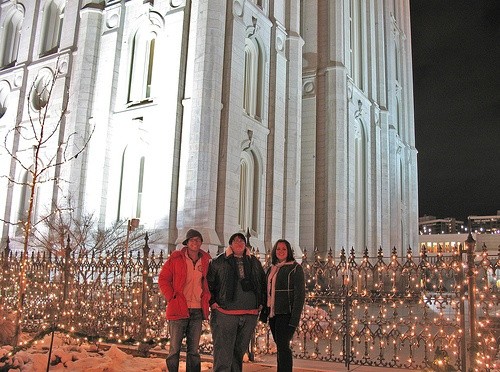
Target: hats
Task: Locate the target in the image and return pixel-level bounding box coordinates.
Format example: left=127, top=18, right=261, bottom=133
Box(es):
left=182, top=229, right=202, bottom=245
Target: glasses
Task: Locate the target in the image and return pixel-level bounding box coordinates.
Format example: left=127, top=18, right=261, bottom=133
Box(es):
left=189, top=238, right=201, bottom=242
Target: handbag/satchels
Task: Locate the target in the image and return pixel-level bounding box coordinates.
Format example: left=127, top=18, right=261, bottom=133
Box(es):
left=240, top=279, right=256, bottom=291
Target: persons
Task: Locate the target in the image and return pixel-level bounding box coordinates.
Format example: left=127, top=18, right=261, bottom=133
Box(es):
left=259, top=239, right=306, bottom=372
left=157, top=229, right=212, bottom=372
left=206, top=233, right=266, bottom=372
